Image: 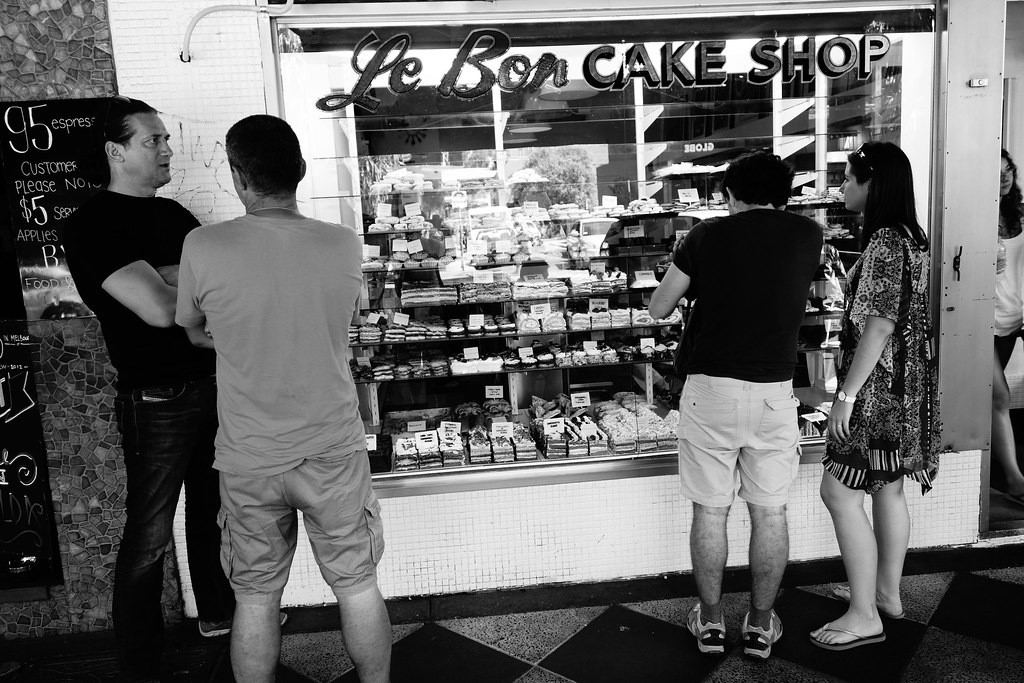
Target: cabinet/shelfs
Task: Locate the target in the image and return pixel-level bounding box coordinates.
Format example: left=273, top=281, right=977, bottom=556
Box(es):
left=310, top=118, right=867, bottom=384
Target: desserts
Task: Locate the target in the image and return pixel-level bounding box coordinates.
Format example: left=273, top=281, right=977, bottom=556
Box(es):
left=348, top=192, right=848, bottom=472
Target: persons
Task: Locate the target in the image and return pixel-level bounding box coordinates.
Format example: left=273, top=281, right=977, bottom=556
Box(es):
left=810, top=143, right=942, bottom=649
left=60, top=96, right=288, bottom=683
left=175, top=114, right=392, bottom=683
left=648, top=151, right=822, bottom=659
left=993, top=148, right=1024, bottom=502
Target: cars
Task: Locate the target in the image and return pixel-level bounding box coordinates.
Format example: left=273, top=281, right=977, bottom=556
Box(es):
left=19, top=266, right=97, bottom=321
left=377, top=204, right=707, bottom=278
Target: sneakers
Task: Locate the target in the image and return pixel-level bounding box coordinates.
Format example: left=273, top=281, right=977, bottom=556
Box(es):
left=686, top=602, right=726, bottom=653
left=740, top=606, right=783, bottom=659
left=198, top=612, right=287, bottom=637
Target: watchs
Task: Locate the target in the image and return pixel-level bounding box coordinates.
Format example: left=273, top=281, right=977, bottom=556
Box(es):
left=838, top=391, right=856, bottom=404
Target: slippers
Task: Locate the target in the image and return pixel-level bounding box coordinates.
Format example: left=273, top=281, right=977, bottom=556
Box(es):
left=844, top=590, right=904, bottom=619
left=809, top=621, right=887, bottom=650
left=1004, top=492, right=1024, bottom=505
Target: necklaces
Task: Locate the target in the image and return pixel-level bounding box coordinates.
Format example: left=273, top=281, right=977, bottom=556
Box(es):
left=251, top=206, right=296, bottom=212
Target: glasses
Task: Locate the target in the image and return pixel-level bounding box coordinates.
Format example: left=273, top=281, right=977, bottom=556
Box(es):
left=1001, top=165, right=1014, bottom=177
